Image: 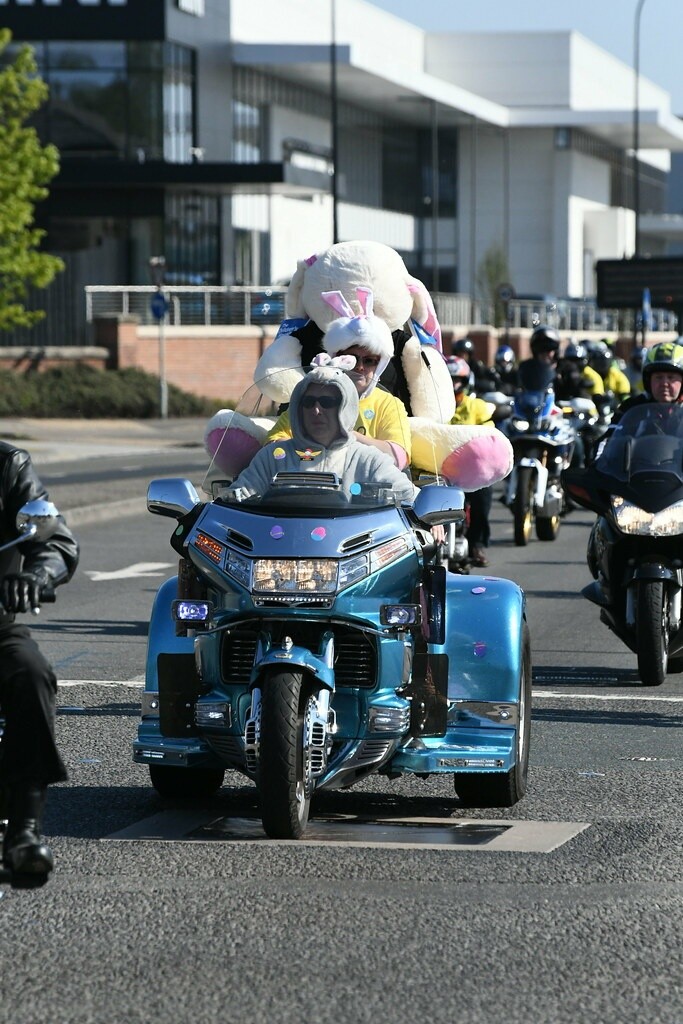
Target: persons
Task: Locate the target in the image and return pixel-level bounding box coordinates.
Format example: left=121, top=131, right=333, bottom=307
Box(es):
left=264, top=345, right=412, bottom=471
left=0, top=441, right=80, bottom=889
left=446, top=328, right=683, bottom=565
left=218, top=353, right=447, bottom=548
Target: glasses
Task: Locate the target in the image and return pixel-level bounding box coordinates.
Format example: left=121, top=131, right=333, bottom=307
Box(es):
left=340, top=353, right=380, bottom=366
left=300, top=395, right=342, bottom=409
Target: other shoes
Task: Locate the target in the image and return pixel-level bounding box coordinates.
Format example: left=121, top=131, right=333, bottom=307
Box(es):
left=471, top=547, right=490, bottom=567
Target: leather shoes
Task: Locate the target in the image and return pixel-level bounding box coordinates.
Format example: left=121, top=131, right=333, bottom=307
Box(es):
left=2, top=812, right=55, bottom=876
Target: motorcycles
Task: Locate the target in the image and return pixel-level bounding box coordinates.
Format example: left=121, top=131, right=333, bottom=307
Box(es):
left=0, top=497, right=64, bottom=834
left=559, top=401, right=683, bottom=686
left=498, top=371, right=576, bottom=547
left=431, top=340, right=647, bottom=517
left=439, top=403, right=513, bottom=577
left=129, top=475, right=533, bottom=841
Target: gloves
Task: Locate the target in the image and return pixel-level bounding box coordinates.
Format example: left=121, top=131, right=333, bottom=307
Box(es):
left=0, top=564, right=54, bottom=616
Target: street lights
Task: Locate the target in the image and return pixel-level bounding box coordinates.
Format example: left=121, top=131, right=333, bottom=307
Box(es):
left=147, top=253, right=171, bottom=420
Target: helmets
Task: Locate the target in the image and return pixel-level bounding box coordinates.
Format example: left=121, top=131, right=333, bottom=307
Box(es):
left=564, top=338, right=617, bottom=364
left=444, top=353, right=471, bottom=393
left=529, top=324, right=560, bottom=359
left=642, top=342, right=683, bottom=396
left=450, top=339, right=476, bottom=360
left=495, top=345, right=515, bottom=367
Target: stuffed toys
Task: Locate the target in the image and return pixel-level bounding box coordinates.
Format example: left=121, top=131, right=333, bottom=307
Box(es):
left=204, top=240, right=514, bottom=492
